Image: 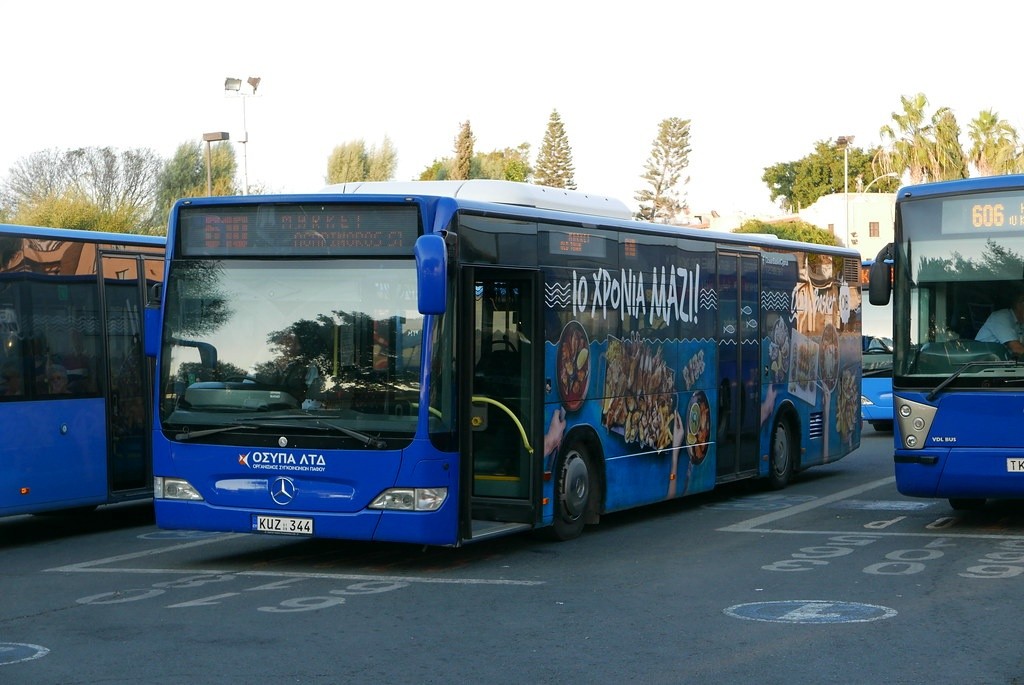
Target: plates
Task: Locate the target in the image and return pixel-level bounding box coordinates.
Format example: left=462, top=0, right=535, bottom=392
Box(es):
left=788, top=328, right=819, bottom=406
left=685, top=391, right=710, bottom=464
left=556, top=321, right=590, bottom=411
left=838, top=368, right=857, bottom=441
left=602, top=334, right=675, bottom=451
left=771, top=320, right=790, bottom=387
left=820, top=324, right=840, bottom=393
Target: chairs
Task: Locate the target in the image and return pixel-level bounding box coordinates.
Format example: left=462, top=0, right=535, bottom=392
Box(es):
left=951, top=286, right=997, bottom=334
left=477, top=338, right=519, bottom=374
left=67, top=355, right=121, bottom=381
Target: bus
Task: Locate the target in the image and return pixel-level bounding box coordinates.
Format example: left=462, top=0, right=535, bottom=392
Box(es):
left=0, top=223, right=166, bottom=519
left=861, top=259, right=895, bottom=431
left=867, top=173, right=1024, bottom=518
left=150, top=179, right=863, bottom=552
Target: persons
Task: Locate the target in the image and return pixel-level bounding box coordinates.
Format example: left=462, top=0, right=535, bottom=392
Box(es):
left=1, top=361, right=22, bottom=395
left=974, top=290, right=1024, bottom=355
left=243, top=326, right=318, bottom=402
left=48, top=364, right=71, bottom=393
left=115, top=333, right=144, bottom=434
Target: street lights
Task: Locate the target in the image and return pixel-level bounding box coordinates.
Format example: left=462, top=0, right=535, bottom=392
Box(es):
left=224, top=73, right=261, bottom=196
